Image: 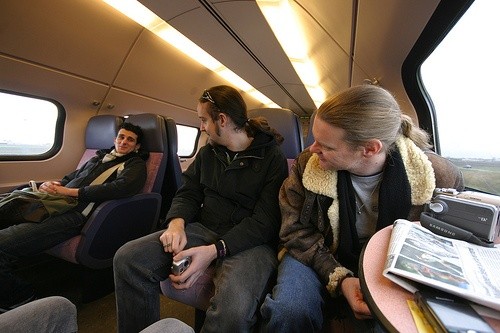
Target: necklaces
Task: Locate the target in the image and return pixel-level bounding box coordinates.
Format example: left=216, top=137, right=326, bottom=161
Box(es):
left=356, top=179, right=381, bottom=215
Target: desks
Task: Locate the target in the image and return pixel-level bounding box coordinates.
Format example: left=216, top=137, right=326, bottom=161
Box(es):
left=357, top=221, right=500, bottom=333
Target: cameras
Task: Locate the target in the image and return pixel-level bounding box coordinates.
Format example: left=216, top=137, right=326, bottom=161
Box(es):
left=170, top=256, right=192, bottom=276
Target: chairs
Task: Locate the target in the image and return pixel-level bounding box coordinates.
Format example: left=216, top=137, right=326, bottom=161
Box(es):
left=25, top=109, right=379, bottom=333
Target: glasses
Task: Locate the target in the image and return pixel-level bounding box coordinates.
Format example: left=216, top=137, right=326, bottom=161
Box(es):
left=200, top=89, right=222, bottom=112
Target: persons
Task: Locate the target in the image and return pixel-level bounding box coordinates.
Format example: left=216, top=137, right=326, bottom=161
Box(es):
left=0, top=122, right=148, bottom=313
left=0, top=296, right=198, bottom=333
left=113, top=85, right=289, bottom=333
left=260, top=84, right=464, bottom=333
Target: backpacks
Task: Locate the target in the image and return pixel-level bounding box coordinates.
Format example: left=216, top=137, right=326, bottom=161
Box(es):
left=0, top=180, right=79, bottom=223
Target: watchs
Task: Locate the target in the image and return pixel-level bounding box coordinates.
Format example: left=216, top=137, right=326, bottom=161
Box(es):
left=215, top=240, right=225, bottom=260
